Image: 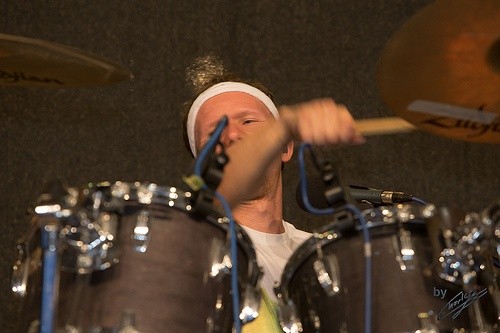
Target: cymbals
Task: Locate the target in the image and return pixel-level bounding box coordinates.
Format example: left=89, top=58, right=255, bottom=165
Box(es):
left=1, top=34, right=134, bottom=85
left=378, top=1, right=498, bottom=141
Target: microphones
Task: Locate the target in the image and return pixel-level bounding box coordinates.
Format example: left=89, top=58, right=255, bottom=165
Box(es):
left=295, top=174, right=412, bottom=213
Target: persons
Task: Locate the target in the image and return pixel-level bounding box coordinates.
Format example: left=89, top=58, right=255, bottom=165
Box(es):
left=180, top=52, right=419, bottom=332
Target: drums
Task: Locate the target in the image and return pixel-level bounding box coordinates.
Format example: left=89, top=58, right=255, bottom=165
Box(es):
left=11, top=182, right=261, bottom=333
left=272, top=204, right=500, bottom=330
